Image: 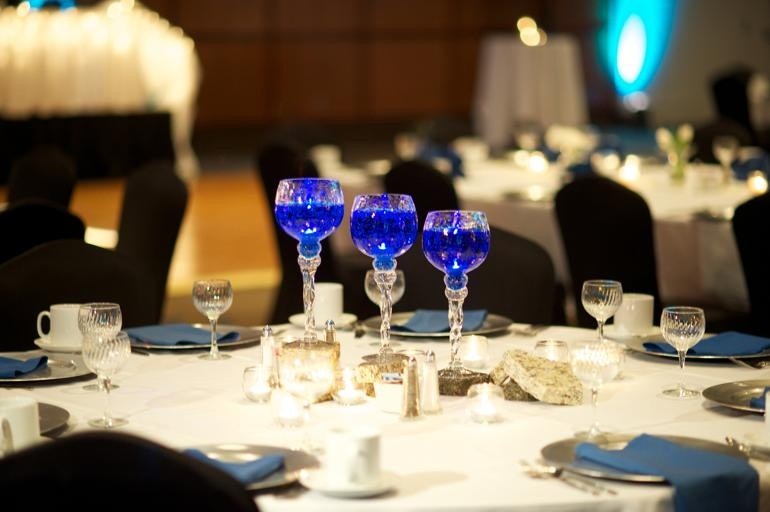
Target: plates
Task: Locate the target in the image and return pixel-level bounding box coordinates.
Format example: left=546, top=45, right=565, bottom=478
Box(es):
left=702, top=377, right=770, bottom=413
left=356, top=308, right=515, bottom=342
left=295, top=465, right=398, bottom=499
left=34, top=338, right=114, bottom=351
left=288, top=311, right=357, bottom=330
left=0, top=351, right=97, bottom=388
left=36, top=402, right=69, bottom=436
left=632, top=332, right=770, bottom=365
left=186, top=443, right=319, bottom=494
left=127, top=323, right=266, bottom=354
left=540, top=433, right=752, bottom=486
left=601, top=322, right=662, bottom=346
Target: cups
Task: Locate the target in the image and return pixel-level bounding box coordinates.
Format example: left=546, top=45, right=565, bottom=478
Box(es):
left=192, top=278, right=233, bottom=364
left=312, top=282, right=343, bottom=322
left=365, top=267, right=406, bottom=367
left=38, top=304, right=91, bottom=343
left=615, top=293, right=652, bottom=336
left=350, top=194, right=418, bottom=375
left=326, top=431, right=379, bottom=486
left=0, top=391, right=40, bottom=452
left=274, top=173, right=346, bottom=357
left=658, top=304, right=707, bottom=403
left=78, top=326, right=135, bottom=435
left=417, top=208, right=492, bottom=384
left=75, top=299, right=124, bottom=396
left=580, top=278, right=624, bottom=354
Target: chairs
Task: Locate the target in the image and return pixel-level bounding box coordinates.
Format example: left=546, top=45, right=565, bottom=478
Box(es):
left=554, top=174, right=666, bottom=328
left=1, top=430, right=260, bottom=511
left=0, top=240, right=161, bottom=357
left=9, top=148, right=77, bottom=214
left=102, top=161, right=192, bottom=325
left=382, top=156, right=458, bottom=229
left=730, top=195, right=770, bottom=338
left=393, top=224, right=565, bottom=331
left=0, top=199, right=85, bottom=263
left=250, top=138, right=379, bottom=326
